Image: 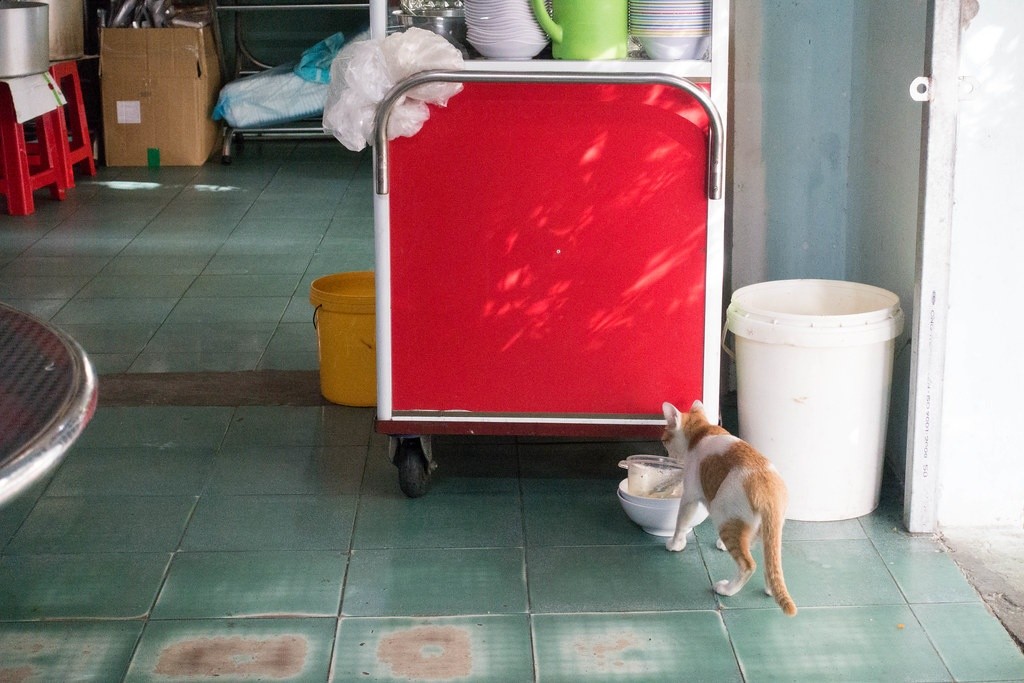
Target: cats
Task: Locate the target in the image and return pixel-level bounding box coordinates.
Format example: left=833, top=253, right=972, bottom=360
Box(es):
left=660, top=399, right=799, bottom=619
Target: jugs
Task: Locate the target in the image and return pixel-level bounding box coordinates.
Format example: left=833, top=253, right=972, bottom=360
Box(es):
left=531, top=0, right=628, bottom=60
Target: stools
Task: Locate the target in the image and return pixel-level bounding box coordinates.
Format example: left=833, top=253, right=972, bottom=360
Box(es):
left=22, top=59, right=95, bottom=191
left=1, top=72, right=67, bottom=216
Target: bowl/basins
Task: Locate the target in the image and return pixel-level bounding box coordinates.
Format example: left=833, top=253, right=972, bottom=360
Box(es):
left=393, top=8, right=466, bottom=46
left=620, top=478, right=706, bottom=506
left=618, top=454, right=687, bottom=499
left=615, top=489, right=713, bottom=536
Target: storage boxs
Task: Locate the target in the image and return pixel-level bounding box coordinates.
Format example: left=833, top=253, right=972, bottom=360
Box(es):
left=97, top=22, right=224, bottom=165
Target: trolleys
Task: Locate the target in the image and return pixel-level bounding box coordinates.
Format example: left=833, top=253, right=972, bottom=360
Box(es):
left=366, top=0, right=728, bottom=500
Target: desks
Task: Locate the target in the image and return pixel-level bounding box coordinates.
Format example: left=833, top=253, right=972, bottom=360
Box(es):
left=1, top=298, right=100, bottom=508
left=214, top=1, right=370, bottom=164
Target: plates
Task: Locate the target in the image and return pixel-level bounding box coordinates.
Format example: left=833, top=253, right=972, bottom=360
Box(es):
left=628, top=1, right=713, bottom=60
left=462, top=0, right=553, bottom=60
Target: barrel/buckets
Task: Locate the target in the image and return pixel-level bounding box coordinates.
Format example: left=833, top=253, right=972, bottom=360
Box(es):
left=0, top=2, right=49, bottom=77
left=722, top=278, right=906, bottom=521
left=310, top=271, right=379, bottom=408
